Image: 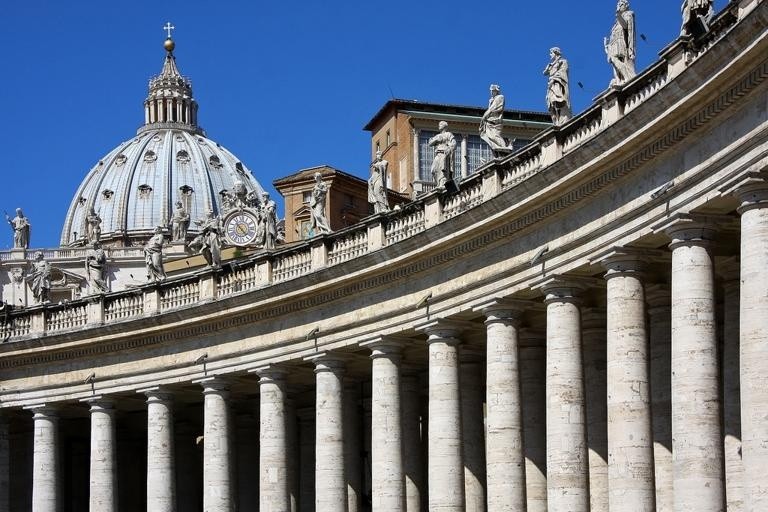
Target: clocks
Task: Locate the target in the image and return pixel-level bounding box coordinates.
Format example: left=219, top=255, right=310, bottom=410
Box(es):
left=224, top=208, right=258, bottom=246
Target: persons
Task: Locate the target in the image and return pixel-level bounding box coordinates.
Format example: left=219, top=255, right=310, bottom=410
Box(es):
left=260, top=192, right=277, bottom=249
left=367, top=151, right=389, bottom=213
left=200, top=209, right=221, bottom=267
left=26, top=251, right=48, bottom=298
left=680, top=0, right=713, bottom=36
left=86, top=240, right=106, bottom=292
left=479, top=84, right=513, bottom=160
left=603, top=0, right=636, bottom=86
left=310, top=172, right=329, bottom=233
left=144, top=226, right=166, bottom=281
left=6, top=208, right=31, bottom=249
left=87, top=208, right=101, bottom=245
left=170, top=201, right=188, bottom=241
left=543, top=47, right=570, bottom=125
left=428, top=121, right=457, bottom=187
